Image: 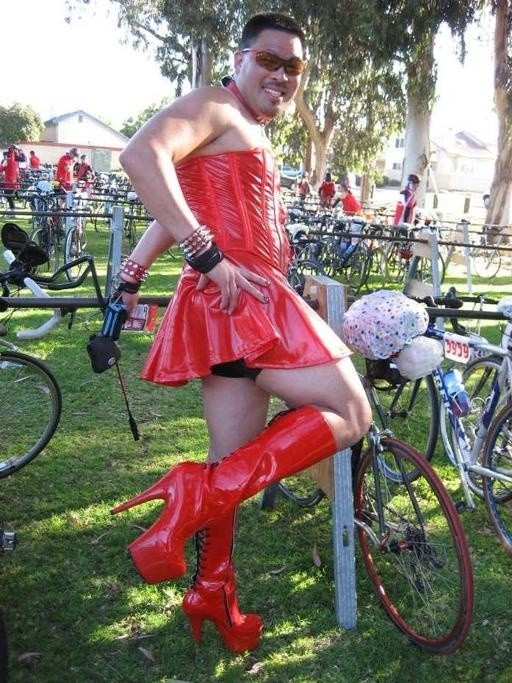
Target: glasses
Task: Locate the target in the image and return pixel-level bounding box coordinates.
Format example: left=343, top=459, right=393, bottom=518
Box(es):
left=238, top=49, right=307, bottom=75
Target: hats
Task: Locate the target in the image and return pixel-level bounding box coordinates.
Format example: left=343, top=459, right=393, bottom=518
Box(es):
left=339, top=288, right=430, bottom=362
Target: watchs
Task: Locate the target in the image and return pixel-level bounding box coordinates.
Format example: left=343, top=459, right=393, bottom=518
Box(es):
left=111, top=273, right=140, bottom=295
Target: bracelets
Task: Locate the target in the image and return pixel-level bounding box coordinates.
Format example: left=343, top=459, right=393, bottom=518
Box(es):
left=118, top=258, right=152, bottom=282
left=185, top=240, right=224, bottom=274
left=181, top=224, right=214, bottom=258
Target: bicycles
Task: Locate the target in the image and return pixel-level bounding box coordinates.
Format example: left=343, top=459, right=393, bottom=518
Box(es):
left=7, top=166, right=152, bottom=288
left=363, top=288, right=512, bottom=558
left=264, top=341, right=474, bottom=657
left=1, top=221, right=101, bottom=480
left=273, top=155, right=511, bottom=297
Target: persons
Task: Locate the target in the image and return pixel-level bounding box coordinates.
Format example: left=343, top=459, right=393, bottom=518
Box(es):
left=0, top=142, right=95, bottom=222
left=375, top=172, right=422, bottom=271
left=482, top=193, right=491, bottom=209
left=318, top=172, right=334, bottom=210
left=105, top=10, right=377, bottom=658
left=330, top=179, right=363, bottom=218
left=296, top=170, right=311, bottom=210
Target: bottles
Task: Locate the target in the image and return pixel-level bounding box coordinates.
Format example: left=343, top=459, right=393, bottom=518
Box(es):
left=442, top=368, right=470, bottom=417
